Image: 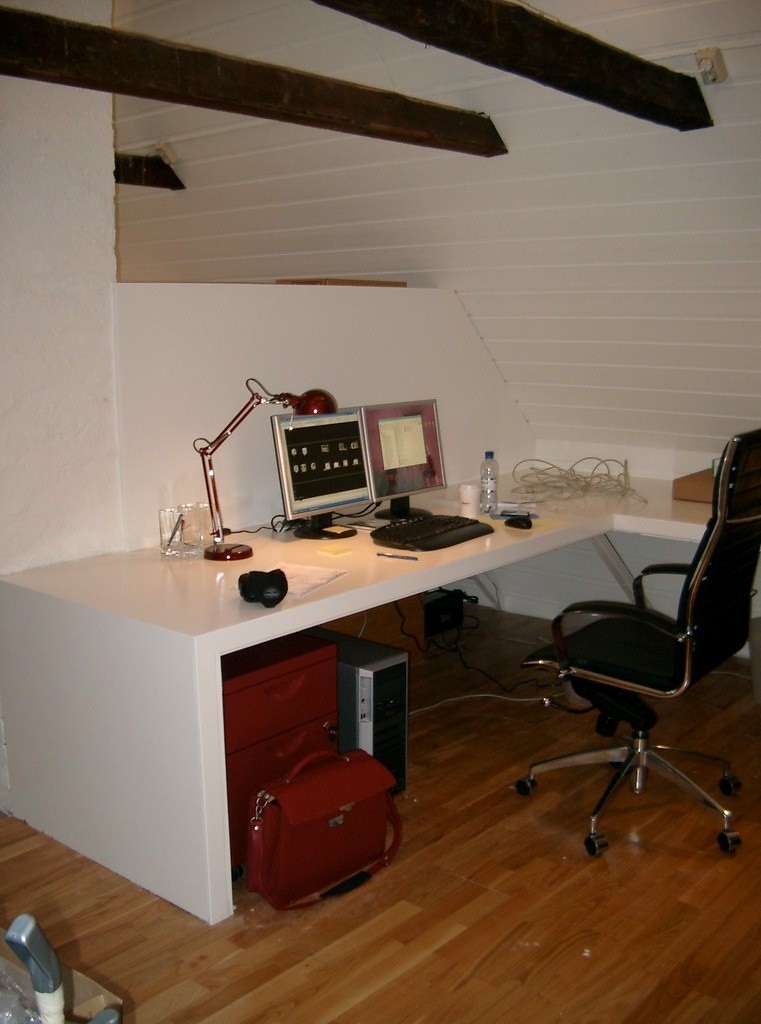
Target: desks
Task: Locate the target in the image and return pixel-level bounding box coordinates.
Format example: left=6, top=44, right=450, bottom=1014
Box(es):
left=0, top=474, right=761, bottom=930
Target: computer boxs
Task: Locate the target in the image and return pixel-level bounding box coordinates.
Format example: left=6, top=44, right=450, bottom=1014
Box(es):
left=305, top=627, right=409, bottom=795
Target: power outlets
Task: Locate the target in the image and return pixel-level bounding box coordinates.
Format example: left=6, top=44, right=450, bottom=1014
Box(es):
left=696, top=47, right=729, bottom=86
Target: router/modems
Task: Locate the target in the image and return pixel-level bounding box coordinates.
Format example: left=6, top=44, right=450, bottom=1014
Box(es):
left=423, top=589, right=465, bottom=638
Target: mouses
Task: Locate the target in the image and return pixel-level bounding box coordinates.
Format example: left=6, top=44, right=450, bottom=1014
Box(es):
left=504, top=516, right=532, bottom=531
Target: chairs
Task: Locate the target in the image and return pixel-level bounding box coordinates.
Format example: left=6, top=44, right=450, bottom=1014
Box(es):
left=515, top=429, right=761, bottom=860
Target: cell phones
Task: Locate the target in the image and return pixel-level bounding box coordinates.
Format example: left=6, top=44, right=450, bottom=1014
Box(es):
left=501, top=511, right=529, bottom=517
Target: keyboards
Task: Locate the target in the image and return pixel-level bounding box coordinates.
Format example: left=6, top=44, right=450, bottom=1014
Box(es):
left=371, top=515, right=494, bottom=552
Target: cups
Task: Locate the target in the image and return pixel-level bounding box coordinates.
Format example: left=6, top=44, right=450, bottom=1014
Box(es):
left=458, top=484, right=482, bottom=520
left=158, top=506, right=203, bottom=557
left=178, top=503, right=212, bottom=556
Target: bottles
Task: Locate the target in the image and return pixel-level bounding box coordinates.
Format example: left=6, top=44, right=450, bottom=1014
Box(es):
left=480, top=450, right=498, bottom=513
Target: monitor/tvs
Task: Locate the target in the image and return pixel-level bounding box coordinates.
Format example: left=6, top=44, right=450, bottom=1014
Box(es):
left=271, top=406, right=373, bottom=540
left=360, top=400, right=447, bottom=521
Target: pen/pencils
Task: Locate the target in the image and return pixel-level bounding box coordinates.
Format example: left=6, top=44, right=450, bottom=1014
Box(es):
left=377, top=552, right=418, bottom=560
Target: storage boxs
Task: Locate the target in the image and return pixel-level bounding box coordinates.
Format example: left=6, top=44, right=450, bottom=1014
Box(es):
left=0, top=927, right=124, bottom=1024
left=672, top=469, right=716, bottom=503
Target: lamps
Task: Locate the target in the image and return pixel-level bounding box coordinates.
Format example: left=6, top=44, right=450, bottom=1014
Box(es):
left=193, top=378, right=337, bottom=560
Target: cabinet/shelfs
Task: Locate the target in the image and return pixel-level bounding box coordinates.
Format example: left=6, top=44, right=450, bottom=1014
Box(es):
left=218, top=628, right=341, bottom=878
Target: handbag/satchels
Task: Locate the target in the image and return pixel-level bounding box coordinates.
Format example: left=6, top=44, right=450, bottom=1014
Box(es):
left=246, top=748, right=403, bottom=910
